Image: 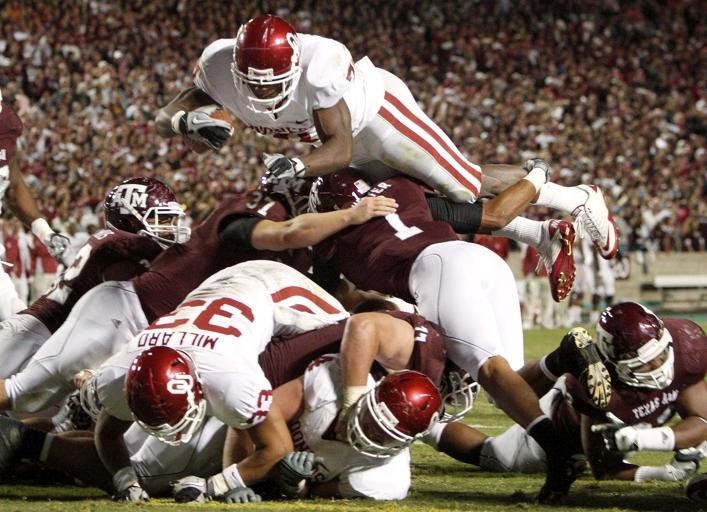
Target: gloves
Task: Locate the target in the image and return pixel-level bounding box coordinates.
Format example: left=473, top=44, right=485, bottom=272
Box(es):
left=522, top=158, right=550, bottom=184
left=634, top=464, right=685, bottom=482
left=590, top=412, right=638, bottom=450
left=261, top=152, right=305, bottom=178
left=279, top=451, right=323, bottom=486
left=43, top=233, right=70, bottom=260
left=119, top=482, right=149, bottom=501
left=179, top=105, right=234, bottom=152
left=169, top=475, right=262, bottom=503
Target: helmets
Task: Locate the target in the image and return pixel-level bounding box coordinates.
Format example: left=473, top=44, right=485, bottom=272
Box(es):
left=374, top=359, right=466, bottom=438
left=258, top=167, right=371, bottom=218
left=104, top=177, right=177, bottom=232
left=231, top=14, right=303, bottom=80
left=596, top=301, right=663, bottom=371
left=126, top=345, right=202, bottom=426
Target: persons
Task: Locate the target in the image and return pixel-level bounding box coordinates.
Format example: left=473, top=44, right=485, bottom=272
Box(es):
left=0, top=0, right=707, bottom=510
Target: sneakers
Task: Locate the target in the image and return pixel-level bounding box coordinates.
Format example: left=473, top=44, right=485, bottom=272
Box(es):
left=0, top=414, right=24, bottom=474
left=570, top=184, right=620, bottom=260
left=539, top=448, right=586, bottom=504
left=674, top=446, right=702, bottom=473
left=537, top=219, right=576, bottom=302
left=561, top=327, right=612, bottom=409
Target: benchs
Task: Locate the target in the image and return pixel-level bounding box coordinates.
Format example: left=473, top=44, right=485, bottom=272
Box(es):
left=643, top=275, right=707, bottom=313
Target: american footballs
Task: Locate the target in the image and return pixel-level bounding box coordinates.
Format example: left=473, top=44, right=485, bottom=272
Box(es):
left=181, top=108, right=229, bottom=155
